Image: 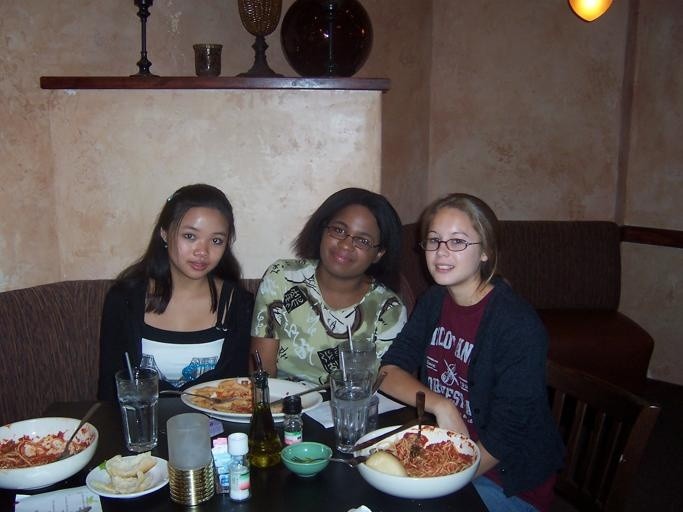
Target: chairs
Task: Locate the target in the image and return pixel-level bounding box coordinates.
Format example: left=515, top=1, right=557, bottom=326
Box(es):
left=548, top=366, right=662, bottom=512
left=388, top=218, right=655, bottom=384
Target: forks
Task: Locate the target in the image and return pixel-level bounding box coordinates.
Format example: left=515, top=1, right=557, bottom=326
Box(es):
left=409, top=390, right=425, bottom=459
left=159, top=390, right=239, bottom=403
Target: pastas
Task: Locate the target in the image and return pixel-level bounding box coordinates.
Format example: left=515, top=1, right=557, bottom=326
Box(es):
left=387, top=438, right=470, bottom=476
left=0, top=439, right=31, bottom=466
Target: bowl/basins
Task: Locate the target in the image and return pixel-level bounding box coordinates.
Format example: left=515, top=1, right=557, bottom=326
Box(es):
left=0, top=415, right=100, bottom=490
left=278, top=442, right=334, bottom=478
left=353, top=426, right=482, bottom=501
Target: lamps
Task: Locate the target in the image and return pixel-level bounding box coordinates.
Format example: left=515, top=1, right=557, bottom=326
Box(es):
left=568, top=0, right=615, bottom=22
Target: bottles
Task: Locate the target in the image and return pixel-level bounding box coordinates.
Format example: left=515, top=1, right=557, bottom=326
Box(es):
left=249, top=349, right=303, bottom=468
left=167, top=413, right=253, bottom=507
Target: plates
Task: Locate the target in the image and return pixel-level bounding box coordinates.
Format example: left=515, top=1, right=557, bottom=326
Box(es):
left=182, top=375, right=324, bottom=424
left=86, top=455, right=173, bottom=500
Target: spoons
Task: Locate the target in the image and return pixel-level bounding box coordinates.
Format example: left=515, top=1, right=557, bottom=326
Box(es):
left=47, top=402, right=102, bottom=464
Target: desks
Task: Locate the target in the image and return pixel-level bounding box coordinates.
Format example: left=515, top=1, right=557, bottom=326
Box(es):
left=39, top=74, right=394, bottom=283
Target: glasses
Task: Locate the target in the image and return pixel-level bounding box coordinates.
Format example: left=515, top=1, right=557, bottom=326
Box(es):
left=325, top=225, right=380, bottom=250
left=418, top=237, right=482, bottom=252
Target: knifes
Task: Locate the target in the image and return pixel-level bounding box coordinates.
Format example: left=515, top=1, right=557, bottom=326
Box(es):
left=350, top=414, right=430, bottom=455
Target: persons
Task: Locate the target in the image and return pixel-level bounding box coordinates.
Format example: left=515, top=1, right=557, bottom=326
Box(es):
left=95, top=183, right=255, bottom=403
left=376, top=192, right=569, bottom=512
left=247, top=187, right=408, bottom=401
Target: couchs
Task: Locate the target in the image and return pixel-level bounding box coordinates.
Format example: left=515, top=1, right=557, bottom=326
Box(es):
left=1, top=271, right=418, bottom=425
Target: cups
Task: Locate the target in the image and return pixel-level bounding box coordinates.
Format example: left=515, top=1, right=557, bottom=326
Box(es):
left=329, top=340, right=378, bottom=453
left=114, top=367, right=159, bottom=452
